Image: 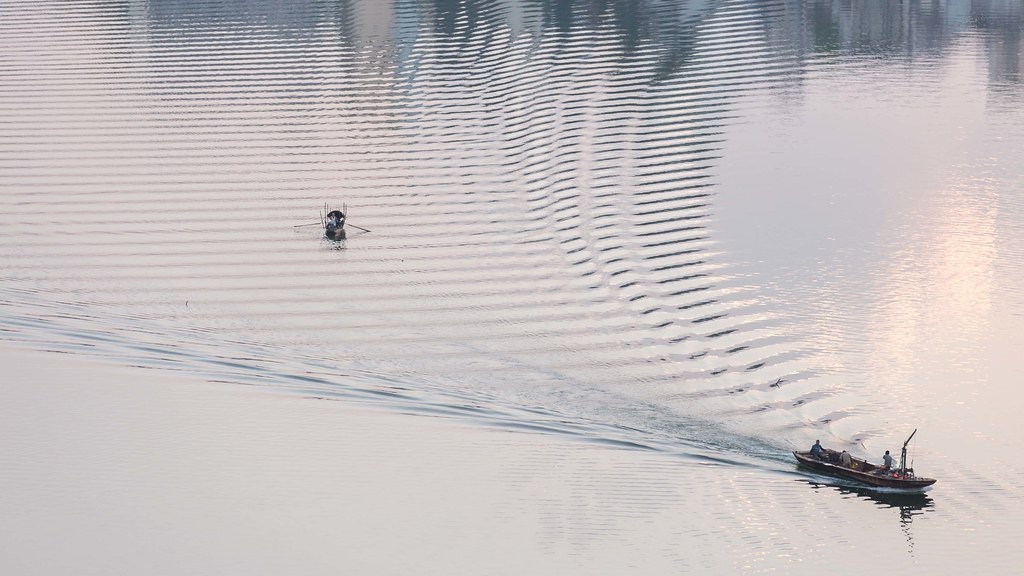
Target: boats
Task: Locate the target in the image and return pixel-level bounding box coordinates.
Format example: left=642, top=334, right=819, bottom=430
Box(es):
left=319, top=201, right=349, bottom=241
left=792, top=428, right=937, bottom=496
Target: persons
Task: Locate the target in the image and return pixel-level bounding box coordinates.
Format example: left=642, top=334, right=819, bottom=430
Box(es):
left=883, top=451, right=892, bottom=470
left=839, top=450, right=853, bottom=469
left=810, top=440, right=826, bottom=458
left=330, top=213, right=341, bottom=227
